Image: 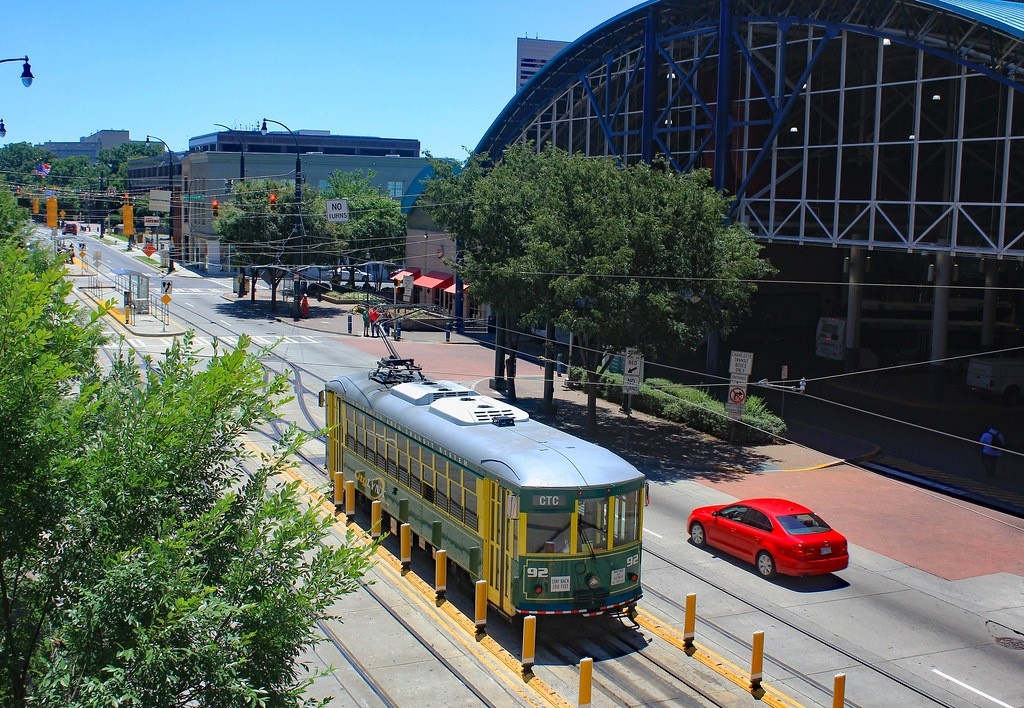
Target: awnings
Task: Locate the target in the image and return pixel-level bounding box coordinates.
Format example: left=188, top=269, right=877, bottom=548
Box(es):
left=389, top=267, right=472, bottom=295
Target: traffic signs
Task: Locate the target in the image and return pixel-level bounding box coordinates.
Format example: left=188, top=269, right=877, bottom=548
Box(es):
left=625, top=347, right=641, bottom=376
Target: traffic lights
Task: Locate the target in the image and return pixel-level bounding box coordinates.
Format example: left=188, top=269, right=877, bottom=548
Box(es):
left=270, top=194, right=277, bottom=212
left=211, top=199, right=219, bottom=219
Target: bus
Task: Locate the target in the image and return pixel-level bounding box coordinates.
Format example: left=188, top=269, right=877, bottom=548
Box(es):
left=836, top=297, right=1016, bottom=326
left=966, top=355, right=1024, bottom=404
left=813, top=317, right=1023, bottom=370
left=316, top=304, right=651, bottom=644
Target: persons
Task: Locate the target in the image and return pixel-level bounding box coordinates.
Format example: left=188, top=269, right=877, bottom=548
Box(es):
left=301, top=293, right=309, bottom=316
left=979, top=424, right=1006, bottom=478
left=362, top=306, right=370, bottom=337
left=381, top=308, right=394, bottom=336
left=62, top=242, right=76, bottom=265
left=369, top=306, right=380, bottom=337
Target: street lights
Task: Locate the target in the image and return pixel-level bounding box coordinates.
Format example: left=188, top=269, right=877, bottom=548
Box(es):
left=92, top=163, right=104, bottom=239
left=108, top=159, right=132, bottom=252
left=261, top=118, right=302, bottom=322
left=145, top=135, right=176, bottom=276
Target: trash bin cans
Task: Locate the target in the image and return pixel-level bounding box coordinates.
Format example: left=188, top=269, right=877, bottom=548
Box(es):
left=233, top=276, right=240, bottom=294
left=245, top=276, right=250, bottom=293
left=136, top=298, right=150, bottom=314
left=557, top=353, right=567, bottom=373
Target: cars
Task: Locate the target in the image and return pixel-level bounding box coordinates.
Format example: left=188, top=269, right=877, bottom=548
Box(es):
left=62, top=223, right=78, bottom=236
left=686, top=497, right=849, bottom=581
left=326, top=267, right=373, bottom=282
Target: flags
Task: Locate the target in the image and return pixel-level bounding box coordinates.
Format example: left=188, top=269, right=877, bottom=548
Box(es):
left=35, top=162, right=52, bottom=179
